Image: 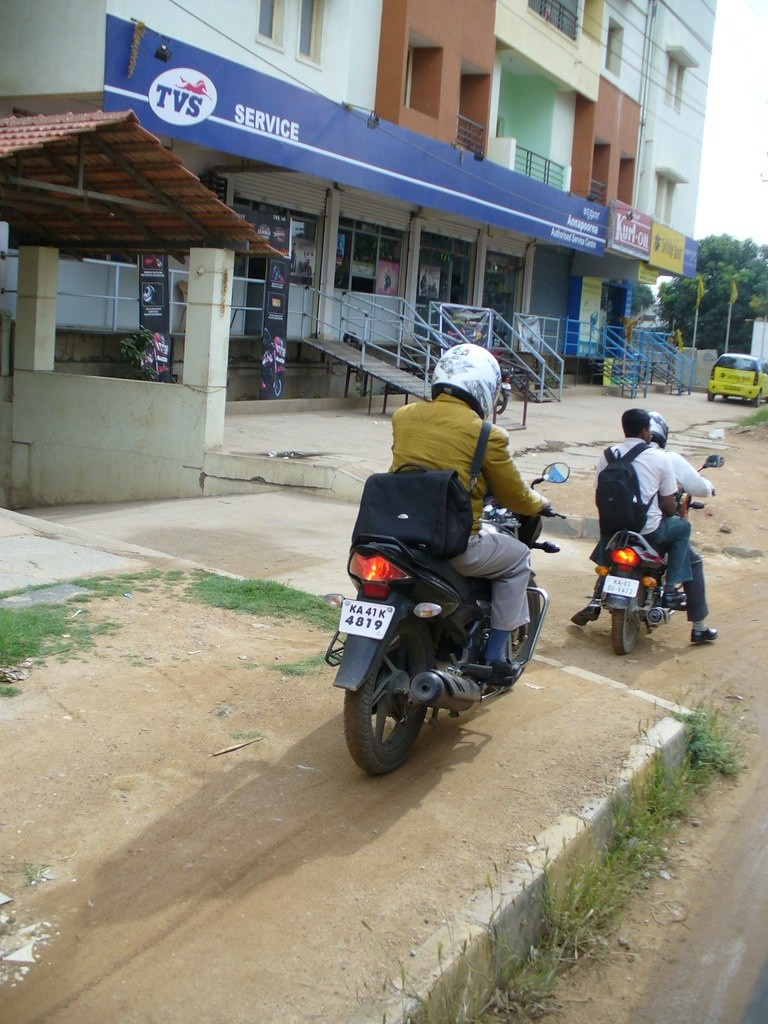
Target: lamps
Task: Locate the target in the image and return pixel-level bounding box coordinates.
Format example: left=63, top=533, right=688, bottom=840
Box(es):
left=569, top=191, right=598, bottom=202
left=343, top=102, right=379, bottom=129
left=608, top=206, right=633, bottom=220
left=132, top=17, right=173, bottom=63
left=456, top=139, right=484, bottom=161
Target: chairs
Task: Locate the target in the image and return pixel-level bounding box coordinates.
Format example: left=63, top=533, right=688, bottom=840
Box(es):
left=178, top=280, right=189, bottom=332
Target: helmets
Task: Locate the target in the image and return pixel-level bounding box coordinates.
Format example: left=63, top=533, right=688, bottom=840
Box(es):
left=431, top=343, right=502, bottom=420
left=647, top=412, right=669, bottom=449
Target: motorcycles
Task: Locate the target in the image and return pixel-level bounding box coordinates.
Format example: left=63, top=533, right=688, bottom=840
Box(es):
left=262, top=327, right=287, bottom=400
left=495, top=361, right=515, bottom=417
left=321, top=459, right=573, bottom=776
left=137, top=324, right=174, bottom=387
left=588, top=453, right=727, bottom=654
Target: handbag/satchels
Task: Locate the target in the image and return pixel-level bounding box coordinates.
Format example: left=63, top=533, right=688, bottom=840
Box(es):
left=350, top=463, right=472, bottom=558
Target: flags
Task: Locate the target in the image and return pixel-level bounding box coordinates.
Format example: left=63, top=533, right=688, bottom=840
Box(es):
left=728, top=279, right=738, bottom=306
left=693, top=276, right=705, bottom=308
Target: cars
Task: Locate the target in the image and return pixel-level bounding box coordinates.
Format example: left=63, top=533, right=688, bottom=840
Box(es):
left=707, top=353, right=767, bottom=408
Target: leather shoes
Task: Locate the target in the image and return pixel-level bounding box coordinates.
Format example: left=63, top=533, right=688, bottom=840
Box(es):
left=571, top=603, right=601, bottom=626
left=691, top=627, right=718, bottom=642
left=660, top=589, right=686, bottom=608
left=485, top=655, right=522, bottom=686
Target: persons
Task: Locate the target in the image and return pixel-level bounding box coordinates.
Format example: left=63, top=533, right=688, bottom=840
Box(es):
left=389, top=343, right=552, bottom=683
left=588, top=409, right=692, bottom=604
left=571, top=412, right=719, bottom=643
left=418, top=269, right=430, bottom=292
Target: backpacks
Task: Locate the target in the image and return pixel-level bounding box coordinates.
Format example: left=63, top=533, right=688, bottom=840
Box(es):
left=595, top=441, right=655, bottom=535
